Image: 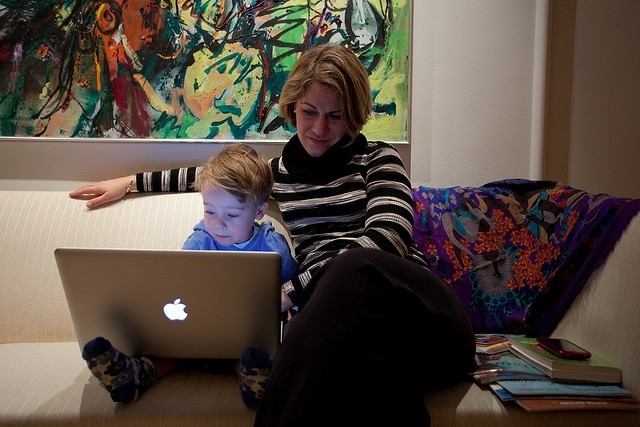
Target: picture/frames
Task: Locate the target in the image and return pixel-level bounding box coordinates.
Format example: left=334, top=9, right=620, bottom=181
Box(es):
left=0, top=0, right=413, bottom=146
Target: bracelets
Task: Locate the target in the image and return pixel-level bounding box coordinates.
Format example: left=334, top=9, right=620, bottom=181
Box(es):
left=125, top=173, right=136, bottom=195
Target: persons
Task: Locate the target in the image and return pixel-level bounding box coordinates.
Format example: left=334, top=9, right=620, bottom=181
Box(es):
left=67, top=45, right=477, bottom=426
left=81, top=143, right=301, bottom=412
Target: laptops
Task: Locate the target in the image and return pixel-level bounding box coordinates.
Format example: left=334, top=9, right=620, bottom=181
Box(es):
left=54, top=246, right=283, bottom=361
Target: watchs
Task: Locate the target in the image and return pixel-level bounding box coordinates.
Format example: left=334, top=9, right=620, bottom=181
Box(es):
left=282, top=279, right=298, bottom=307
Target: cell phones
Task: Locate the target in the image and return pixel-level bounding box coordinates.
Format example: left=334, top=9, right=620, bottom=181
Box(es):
left=536, top=338, right=591, bottom=360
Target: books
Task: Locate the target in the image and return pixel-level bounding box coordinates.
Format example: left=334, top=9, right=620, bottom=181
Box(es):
left=468, top=330, right=640, bottom=416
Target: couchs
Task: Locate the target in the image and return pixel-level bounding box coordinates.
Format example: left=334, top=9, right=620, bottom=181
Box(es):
left=0, top=178, right=640, bottom=426
